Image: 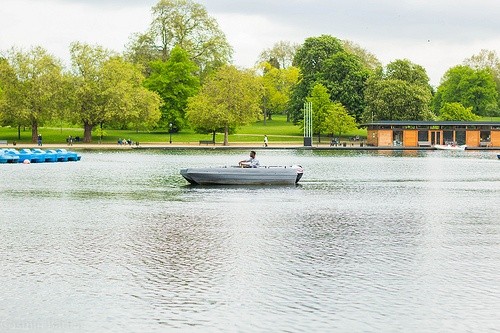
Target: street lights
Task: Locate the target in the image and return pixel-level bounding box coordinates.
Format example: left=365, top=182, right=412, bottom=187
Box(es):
left=169, top=123, right=172, bottom=144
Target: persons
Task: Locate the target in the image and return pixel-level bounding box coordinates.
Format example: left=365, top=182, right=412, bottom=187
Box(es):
left=69, top=136, right=72, bottom=146
left=75, top=136, right=79, bottom=142
left=118, top=139, right=122, bottom=145
left=122, top=139, right=126, bottom=144
left=38, top=134, right=42, bottom=146
left=331, top=139, right=337, bottom=146
left=127, top=138, right=131, bottom=145
left=264, top=135, right=268, bottom=146
left=239, top=151, right=259, bottom=168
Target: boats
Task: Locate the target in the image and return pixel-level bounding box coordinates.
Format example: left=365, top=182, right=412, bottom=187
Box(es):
left=179, top=164, right=304, bottom=185
left=433, top=144, right=468, bottom=151
left=0, top=148, right=82, bottom=163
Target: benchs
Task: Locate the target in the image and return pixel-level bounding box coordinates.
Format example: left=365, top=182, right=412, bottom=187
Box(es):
left=330, top=143, right=364, bottom=147
left=418, top=141, right=431, bottom=147
left=66, top=139, right=83, bottom=144
left=0, top=140, right=7, bottom=145
left=480, top=142, right=491, bottom=147
left=199, top=141, right=215, bottom=145
